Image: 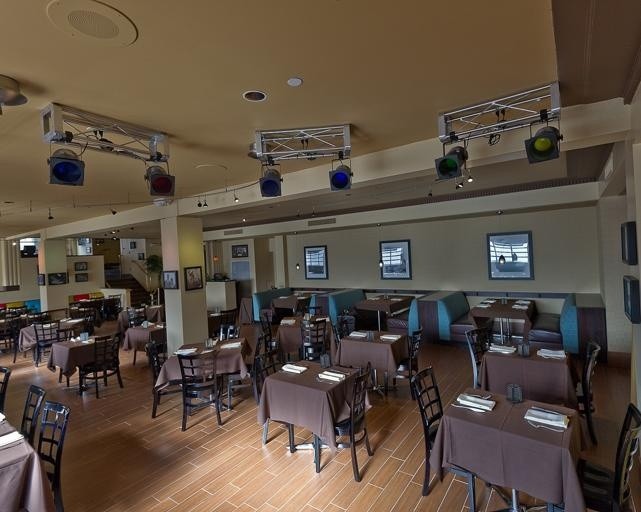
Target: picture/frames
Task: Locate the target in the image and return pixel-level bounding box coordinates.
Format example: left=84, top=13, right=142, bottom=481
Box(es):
left=379, top=240, right=412, bottom=280
left=486, top=229, right=534, bottom=280
left=86, top=237, right=91, bottom=253
left=129, top=241, right=144, bottom=261
left=38, top=262, right=88, bottom=285
left=621, top=221, right=638, bottom=265
left=162, top=265, right=204, bottom=292
left=231, top=244, right=248, bottom=257
left=623, top=275, right=641, bottom=324
left=304, top=245, right=328, bottom=280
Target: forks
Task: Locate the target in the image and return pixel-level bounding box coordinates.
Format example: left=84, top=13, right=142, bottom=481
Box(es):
left=527, top=420, right=563, bottom=434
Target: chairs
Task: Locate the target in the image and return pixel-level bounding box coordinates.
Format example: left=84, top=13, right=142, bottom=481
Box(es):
left=1, top=294, right=148, bottom=511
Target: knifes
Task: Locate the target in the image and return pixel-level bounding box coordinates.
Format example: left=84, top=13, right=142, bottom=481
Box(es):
left=451, top=403, right=486, bottom=414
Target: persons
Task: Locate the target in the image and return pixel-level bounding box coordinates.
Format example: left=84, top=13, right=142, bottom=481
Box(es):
left=196, top=277, right=201, bottom=286
left=187, top=270, right=194, bottom=287
left=164, top=273, right=174, bottom=288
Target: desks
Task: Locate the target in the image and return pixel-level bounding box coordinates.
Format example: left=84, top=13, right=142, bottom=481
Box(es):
left=206, top=280, right=237, bottom=312
